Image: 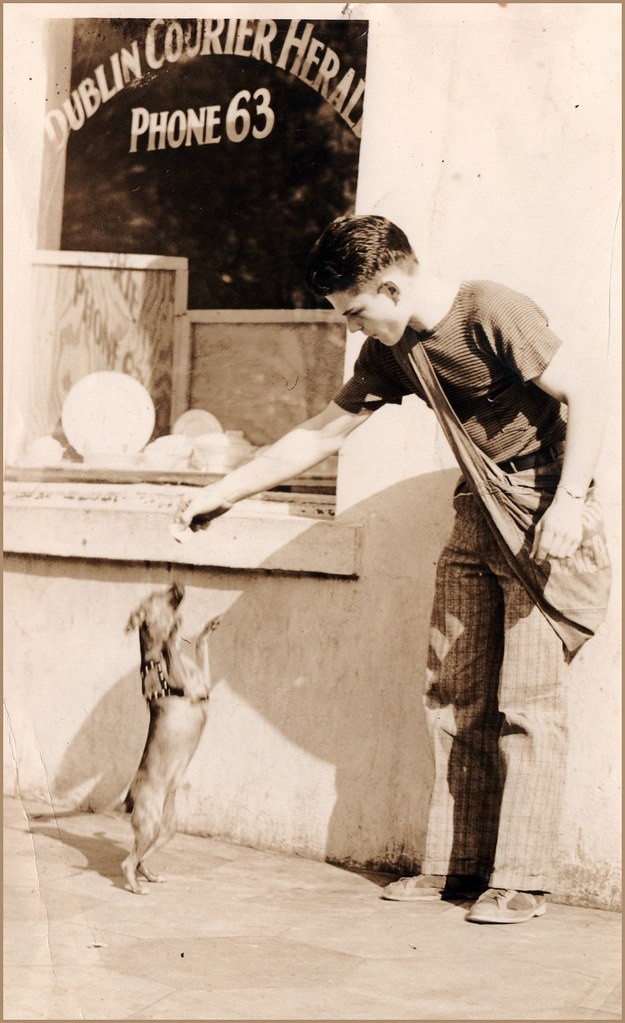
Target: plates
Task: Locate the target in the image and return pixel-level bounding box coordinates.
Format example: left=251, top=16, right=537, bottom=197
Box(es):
left=62, top=371, right=155, bottom=457
left=172, top=409, right=222, bottom=438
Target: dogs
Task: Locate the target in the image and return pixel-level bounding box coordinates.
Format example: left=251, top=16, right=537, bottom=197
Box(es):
left=113, top=578, right=224, bottom=896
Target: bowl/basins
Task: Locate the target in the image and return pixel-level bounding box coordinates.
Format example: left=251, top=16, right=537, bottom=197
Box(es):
left=141, top=430, right=252, bottom=472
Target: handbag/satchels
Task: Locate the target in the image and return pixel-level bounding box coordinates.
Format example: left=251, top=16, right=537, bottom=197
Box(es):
left=464, top=448, right=611, bottom=662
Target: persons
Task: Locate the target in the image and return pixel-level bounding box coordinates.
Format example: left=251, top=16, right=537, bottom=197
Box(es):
left=168, top=215, right=612, bottom=924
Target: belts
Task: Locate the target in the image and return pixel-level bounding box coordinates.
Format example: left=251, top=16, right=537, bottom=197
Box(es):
left=498, top=439, right=566, bottom=474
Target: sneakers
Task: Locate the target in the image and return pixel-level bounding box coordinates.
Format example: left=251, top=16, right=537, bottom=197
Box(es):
left=466, top=888, right=548, bottom=923
left=381, top=872, right=483, bottom=901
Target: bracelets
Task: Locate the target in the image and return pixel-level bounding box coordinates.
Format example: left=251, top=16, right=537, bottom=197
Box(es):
left=556, top=483, right=586, bottom=499
